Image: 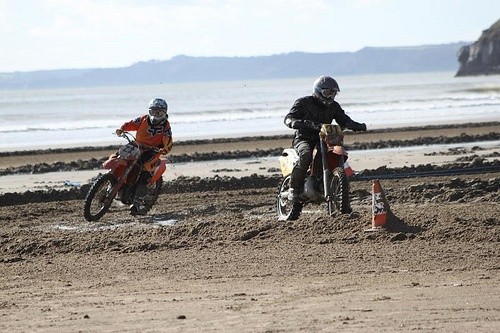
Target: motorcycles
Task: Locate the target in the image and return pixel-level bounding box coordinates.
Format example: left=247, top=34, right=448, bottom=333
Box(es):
left=82, top=131, right=166, bottom=223
left=276, top=122, right=359, bottom=222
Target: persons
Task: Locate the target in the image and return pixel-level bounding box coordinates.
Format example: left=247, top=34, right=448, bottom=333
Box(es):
left=115, top=98, right=173, bottom=202
left=283, top=76, right=366, bottom=201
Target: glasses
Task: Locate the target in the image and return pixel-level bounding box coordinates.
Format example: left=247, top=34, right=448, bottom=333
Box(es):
left=322, top=89, right=336, bottom=99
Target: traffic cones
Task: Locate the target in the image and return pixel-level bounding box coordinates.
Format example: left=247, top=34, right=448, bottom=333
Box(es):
left=344, top=161, right=356, bottom=179
left=361, top=178, right=393, bottom=232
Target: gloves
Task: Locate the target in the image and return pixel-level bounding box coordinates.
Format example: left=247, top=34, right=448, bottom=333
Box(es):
left=293, top=120, right=311, bottom=130
left=347, top=120, right=362, bottom=132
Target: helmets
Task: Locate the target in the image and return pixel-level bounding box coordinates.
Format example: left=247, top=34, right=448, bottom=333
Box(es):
left=148, top=97, right=167, bottom=126
left=313, top=75, right=340, bottom=105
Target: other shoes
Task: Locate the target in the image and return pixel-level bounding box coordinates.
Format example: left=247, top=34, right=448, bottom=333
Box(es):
left=288, top=187, right=299, bottom=200
left=134, top=187, right=153, bottom=201
